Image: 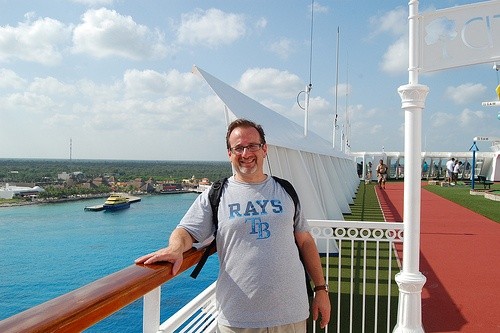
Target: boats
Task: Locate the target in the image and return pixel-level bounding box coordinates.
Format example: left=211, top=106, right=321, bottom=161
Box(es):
left=104, top=194, right=132, bottom=209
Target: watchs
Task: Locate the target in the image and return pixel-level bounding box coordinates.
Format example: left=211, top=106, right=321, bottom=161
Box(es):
left=313, top=283, right=329, bottom=292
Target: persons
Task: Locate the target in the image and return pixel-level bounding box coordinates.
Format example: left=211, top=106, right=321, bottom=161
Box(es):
left=453, top=160, right=458, bottom=183
left=446, top=157, right=455, bottom=186
left=134, top=118, right=332, bottom=333
left=453, top=161, right=463, bottom=185
left=461, top=159, right=470, bottom=178
left=437, top=163, right=442, bottom=177
left=431, top=161, right=437, bottom=177
left=422, top=161, right=429, bottom=177
left=355, top=161, right=401, bottom=180
left=376, top=159, right=388, bottom=189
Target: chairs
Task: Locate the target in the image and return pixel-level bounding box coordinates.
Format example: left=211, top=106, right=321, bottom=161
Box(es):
left=477, top=175, right=494, bottom=189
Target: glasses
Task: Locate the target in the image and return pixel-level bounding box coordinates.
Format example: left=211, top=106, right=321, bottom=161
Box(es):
left=230, top=143, right=264, bottom=153
